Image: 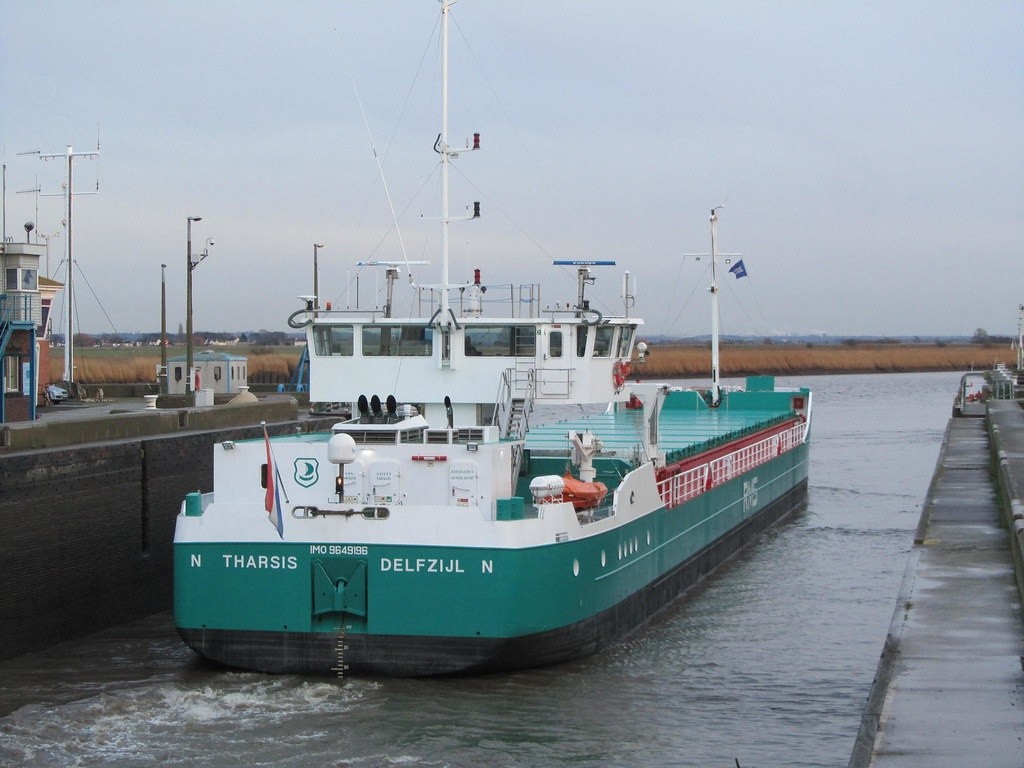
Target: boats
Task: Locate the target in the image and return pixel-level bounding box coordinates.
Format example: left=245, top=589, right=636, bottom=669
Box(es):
left=527, top=469, right=608, bottom=513
left=163, top=1, right=817, bottom=681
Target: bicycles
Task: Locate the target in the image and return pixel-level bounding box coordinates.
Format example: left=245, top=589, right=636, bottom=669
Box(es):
left=38, top=383, right=51, bottom=407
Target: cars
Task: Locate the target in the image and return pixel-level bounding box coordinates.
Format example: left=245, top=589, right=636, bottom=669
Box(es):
left=50, top=386, right=69, bottom=405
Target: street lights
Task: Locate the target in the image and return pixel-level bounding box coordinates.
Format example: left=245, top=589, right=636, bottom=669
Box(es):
left=313, top=244, right=325, bottom=316
left=185, top=214, right=215, bottom=412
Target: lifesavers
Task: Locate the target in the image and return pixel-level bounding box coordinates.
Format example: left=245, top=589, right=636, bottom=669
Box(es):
left=615, top=362, right=626, bottom=386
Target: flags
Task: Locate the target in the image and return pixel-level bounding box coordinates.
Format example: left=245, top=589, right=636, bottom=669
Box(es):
left=728, top=259, right=747, bottom=279
left=264, top=428, right=284, bottom=540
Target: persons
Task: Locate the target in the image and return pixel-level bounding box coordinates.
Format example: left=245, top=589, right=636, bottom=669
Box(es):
left=43, top=386, right=51, bottom=406
left=974, top=390, right=981, bottom=398
